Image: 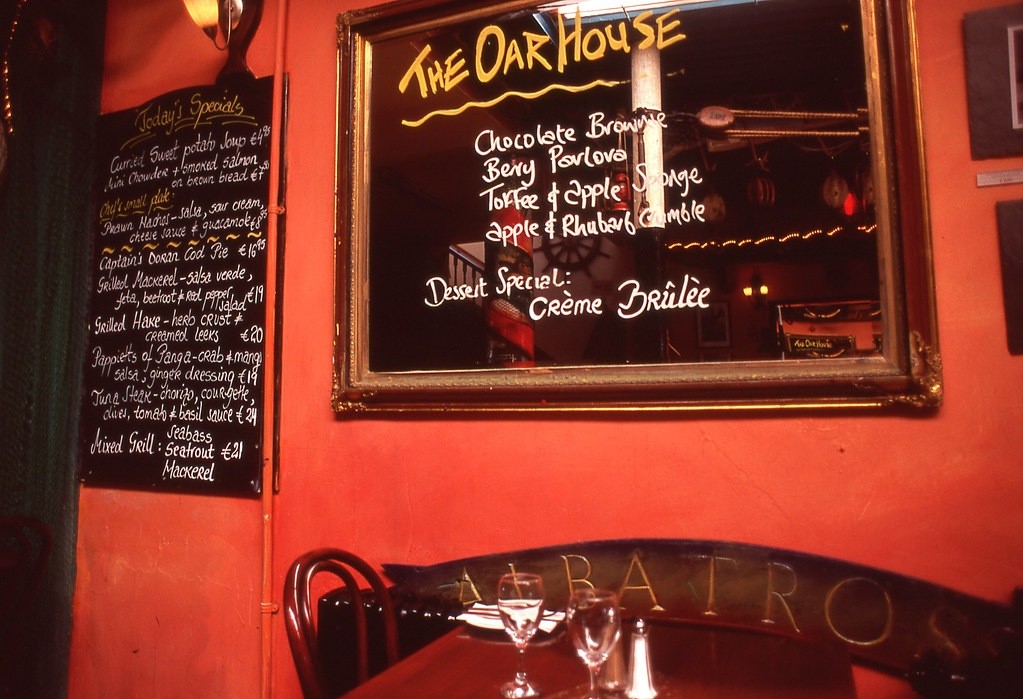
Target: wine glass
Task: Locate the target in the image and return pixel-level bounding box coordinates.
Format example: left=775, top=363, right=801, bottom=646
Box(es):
left=567, top=589, right=621, bottom=699
left=496, top=572, right=545, bottom=698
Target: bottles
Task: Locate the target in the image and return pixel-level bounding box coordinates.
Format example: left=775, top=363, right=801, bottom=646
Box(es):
left=624, top=618, right=659, bottom=699
left=599, top=607, right=628, bottom=692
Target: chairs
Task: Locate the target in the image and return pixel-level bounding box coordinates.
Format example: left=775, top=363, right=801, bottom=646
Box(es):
left=283, top=546, right=398, bottom=699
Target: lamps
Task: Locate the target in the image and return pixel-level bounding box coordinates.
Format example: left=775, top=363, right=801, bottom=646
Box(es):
left=742, top=274, right=768, bottom=310
left=183, top=0, right=262, bottom=102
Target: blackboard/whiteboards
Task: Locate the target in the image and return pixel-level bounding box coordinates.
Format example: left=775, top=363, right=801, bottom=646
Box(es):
left=80, top=73, right=289, bottom=498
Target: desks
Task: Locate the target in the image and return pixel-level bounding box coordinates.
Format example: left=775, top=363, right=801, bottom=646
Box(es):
left=342, top=615, right=855, bottom=699
